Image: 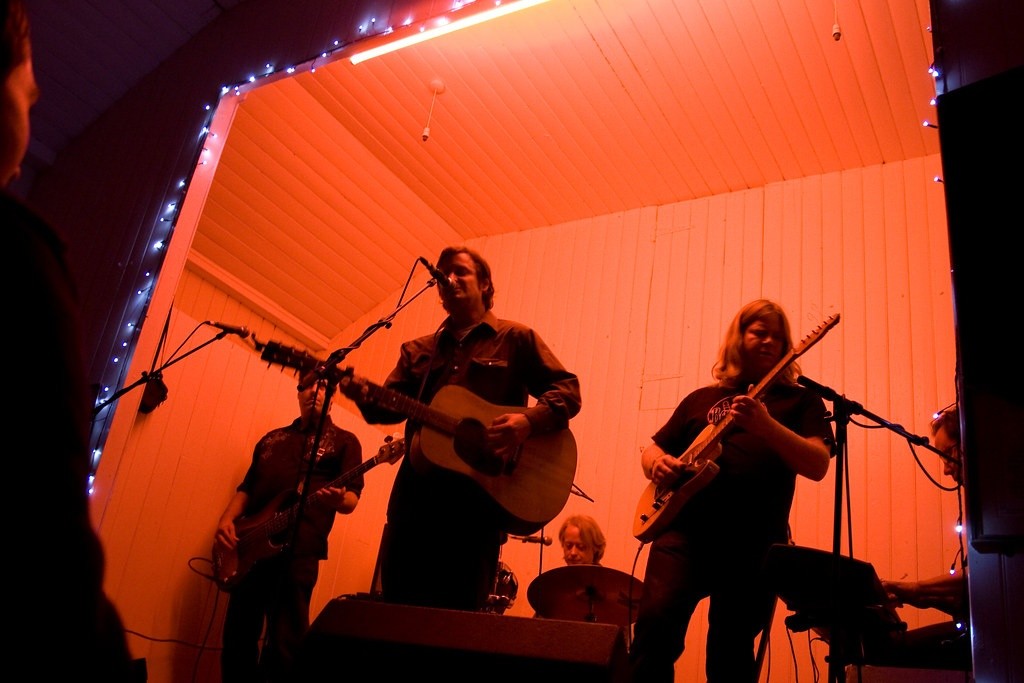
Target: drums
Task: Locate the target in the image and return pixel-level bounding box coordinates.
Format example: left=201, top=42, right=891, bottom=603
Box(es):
left=476, top=559, right=519, bottom=615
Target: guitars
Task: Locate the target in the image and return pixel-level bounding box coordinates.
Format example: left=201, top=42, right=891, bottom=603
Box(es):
left=630, top=311, right=844, bottom=544
left=210, top=434, right=406, bottom=592
left=259, top=336, right=580, bottom=540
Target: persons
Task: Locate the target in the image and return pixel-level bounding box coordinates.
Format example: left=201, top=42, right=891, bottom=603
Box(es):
left=879, top=403, right=969, bottom=669
left=532, top=514, right=606, bottom=619
left=340, top=246, right=582, bottom=608
left=632, top=299, right=837, bottom=683
left=0, top=0, right=152, bottom=683
left=213, top=370, right=363, bottom=683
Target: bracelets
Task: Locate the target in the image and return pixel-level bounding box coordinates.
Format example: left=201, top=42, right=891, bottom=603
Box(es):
left=649, top=453, right=667, bottom=480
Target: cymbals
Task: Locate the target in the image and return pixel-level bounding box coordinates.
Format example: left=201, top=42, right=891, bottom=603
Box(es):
left=525, top=562, right=645, bottom=627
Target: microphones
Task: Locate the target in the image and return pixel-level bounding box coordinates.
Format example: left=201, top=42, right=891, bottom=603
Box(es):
left=205, top=320, right=250, bottom=338
left=522, top=535, right=553, bottom=546
left=419, top=256, right=452, bottom=289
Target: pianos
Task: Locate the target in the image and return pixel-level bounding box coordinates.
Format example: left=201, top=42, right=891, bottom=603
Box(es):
left=775, top=543, right=910, bottom=663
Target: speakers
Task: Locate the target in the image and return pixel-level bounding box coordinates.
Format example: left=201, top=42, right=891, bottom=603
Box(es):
left=292, top=592, right=628, bottom=683
